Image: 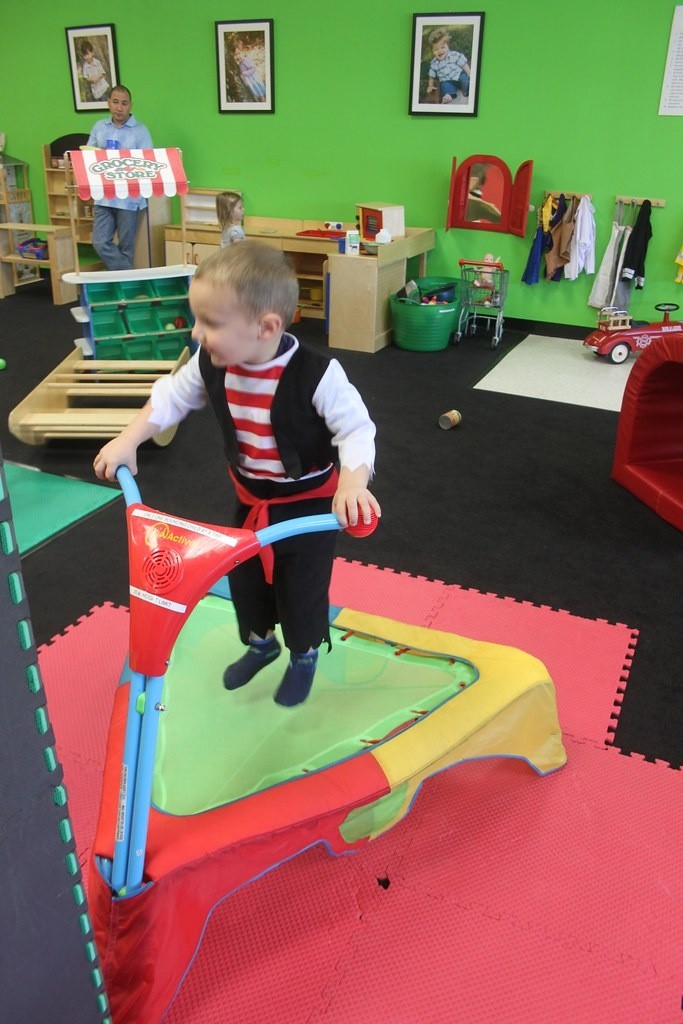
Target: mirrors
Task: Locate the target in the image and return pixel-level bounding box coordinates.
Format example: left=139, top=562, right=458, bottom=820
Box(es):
left=464, top=164, right=504, bottom=225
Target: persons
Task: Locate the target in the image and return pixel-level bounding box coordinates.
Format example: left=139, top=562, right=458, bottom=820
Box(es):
left=94, top=240, right=381, bottom=706
left=474, top=254, right=500, bottom=287
left=233, top=43, right=266, bottom=99
left=426, top=29, right=471, bottom=104
left=81, top=41, right=110, bottom=101
left=216, top=192, right=246, bottom=248
left=86, top=85, right=153, bottom=270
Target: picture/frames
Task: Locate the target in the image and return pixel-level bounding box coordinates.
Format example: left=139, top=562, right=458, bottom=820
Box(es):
left=408, top=10, right=485, bottom=117
left=213, top=18, right=274, bottom=114
left=64, top=23, right=121, bottom=112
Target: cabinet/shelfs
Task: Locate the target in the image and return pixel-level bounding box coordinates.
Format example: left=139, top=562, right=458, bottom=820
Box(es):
left=163, top=220, right=434, bottom=353
left=0, top=222, right=78, bottom=305
left=43, top=133, right=173, bottom=272
left=62, top=265, right=198, bottom=401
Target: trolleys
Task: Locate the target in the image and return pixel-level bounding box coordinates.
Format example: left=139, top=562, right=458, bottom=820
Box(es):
left=454, top=259, right=509, bottom=350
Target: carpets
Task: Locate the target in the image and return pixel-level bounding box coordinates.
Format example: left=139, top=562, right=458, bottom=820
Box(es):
left=472, top=334, right=643, bottom=412
